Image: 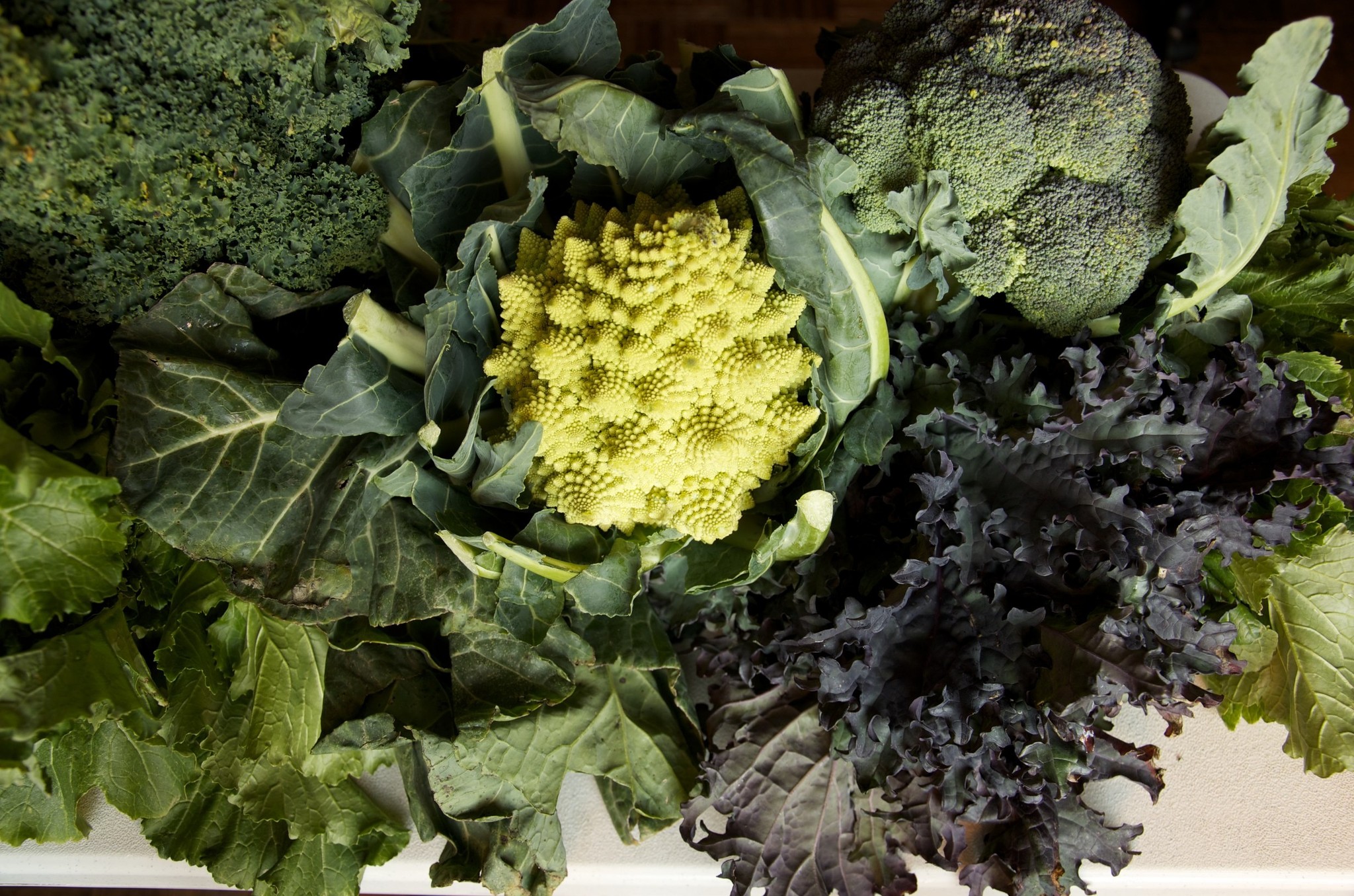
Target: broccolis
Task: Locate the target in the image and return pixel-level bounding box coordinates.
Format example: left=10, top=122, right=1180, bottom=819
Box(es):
left=0, top=0, right=1197, bottom=549
